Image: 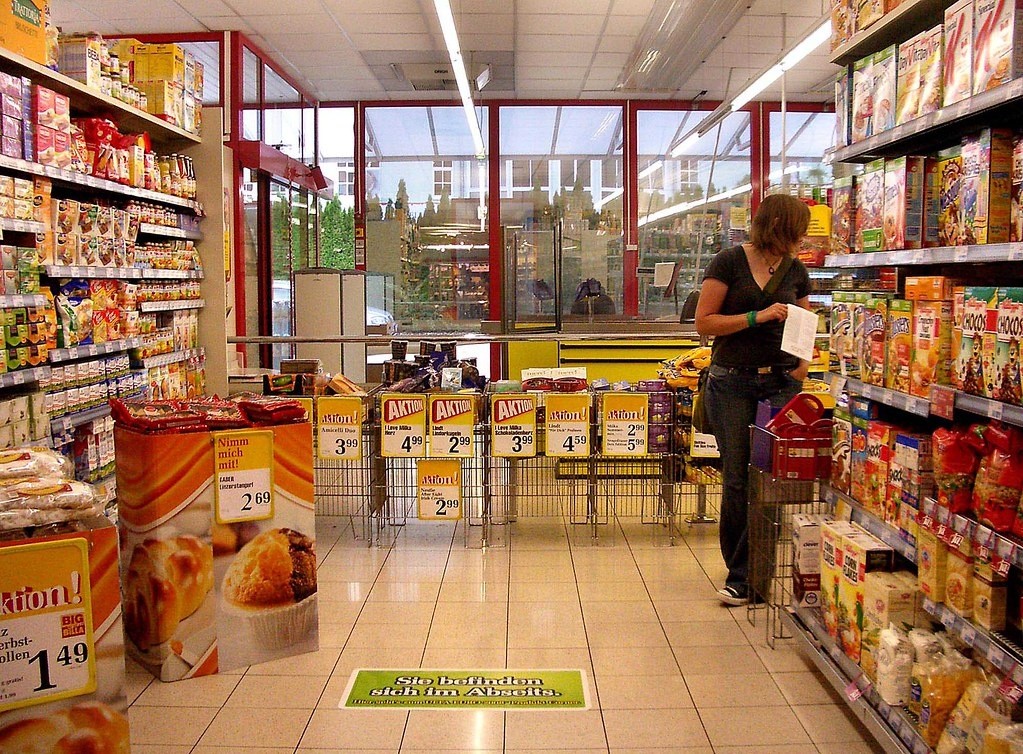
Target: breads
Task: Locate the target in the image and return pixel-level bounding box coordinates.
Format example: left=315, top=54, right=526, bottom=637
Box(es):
left=0, top=700, right=132, bottom=754
left=122, top=532, right=213, bottom=653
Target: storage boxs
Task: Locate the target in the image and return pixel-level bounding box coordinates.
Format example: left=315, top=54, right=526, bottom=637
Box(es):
left=829, top=391, right=939, bottom=539
left=918, top=526, right=1006, bottom=632
left=148, top=355, right=207, bottom=403
left=0, top=71, right=71, bottom=170
left=792, top=513, right=932, bottom=681
left=88, top=278, right=120, bottom=343
left=34, top=177, right=140, bottom=268
left=108, top=38, right=205, bottom=136
left=0, top=391, right=51, bottom=449
left=1, top=0, right=47, bottom=66
left=830, top=276, right=1023, bottom=407
left=0, top=245, right=40, bottom=295
left=0, top=175, right=33, bottom=220
left=58, top=38, right=100, bottom=91
left=830, top=128, right=1023, bottom=254
left=814, top=0, right=1023, bottom=150
left=156, top=309, right=198, bottom=351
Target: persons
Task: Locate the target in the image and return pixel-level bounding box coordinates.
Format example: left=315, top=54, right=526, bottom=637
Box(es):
left=696, top=194, right=810, bottom=606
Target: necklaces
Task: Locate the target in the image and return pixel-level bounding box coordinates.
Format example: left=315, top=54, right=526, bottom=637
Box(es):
left=759, top=248, right=781, bottom=274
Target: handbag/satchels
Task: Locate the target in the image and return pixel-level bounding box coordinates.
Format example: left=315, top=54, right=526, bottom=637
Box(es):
left=690, top=366, right=714, bottom=434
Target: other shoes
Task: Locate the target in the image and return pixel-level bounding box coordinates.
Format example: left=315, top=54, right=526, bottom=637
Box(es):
left=717, top=581, right=762, bottom=607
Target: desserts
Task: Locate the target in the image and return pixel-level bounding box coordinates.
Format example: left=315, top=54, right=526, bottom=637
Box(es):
left=220, top=526, right=317, bottom=648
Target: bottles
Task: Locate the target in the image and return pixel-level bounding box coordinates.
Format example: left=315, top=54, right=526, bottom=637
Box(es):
left=169, top=153, right=182, bottom=197
left=108, top=51, right=120, bottom=75
left=137, top=280, right=182, bottom=303
left=124, top=199, right=177, bottom=228
left=134, top=87, right=140, bottom=110
left=122, top=83, right=128, bottom=104
left=119, top=61, right=124, bottom=83
left=111, top=75, right=119, bottom=100
left=134, top=240, right=173, bottom=269
left=100, top=41, right=110, bottom=72
left=159, top=156, right=172, bottom=195
left=154, top=156, right=161, bottom=193
left=178, top=155, right=188, bottom=200
left=106, top=72, right=112, bottom=96
left=119, top=75, right=122, bottom=101
left=101, top=72, right=106, bottom=95
left=128, top=85, right=134, bottom=107
left=140, top=92, right=147, bottom=113
left=184, top=157, right=192, bottom=199
left=124, top=63, right=129, bottom=85
left=189, top=158, right=196, bottom=201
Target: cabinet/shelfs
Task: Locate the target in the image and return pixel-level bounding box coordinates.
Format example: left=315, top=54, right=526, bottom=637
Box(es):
left=0, top=46, right=202, bottom=525
left=778, top=0, right=1023, bottom=754
left=367, top=220, right=728, bottom=323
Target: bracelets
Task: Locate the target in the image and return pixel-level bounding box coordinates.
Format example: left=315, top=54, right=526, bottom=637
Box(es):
left=747, top=311, right=757, bottom=327
